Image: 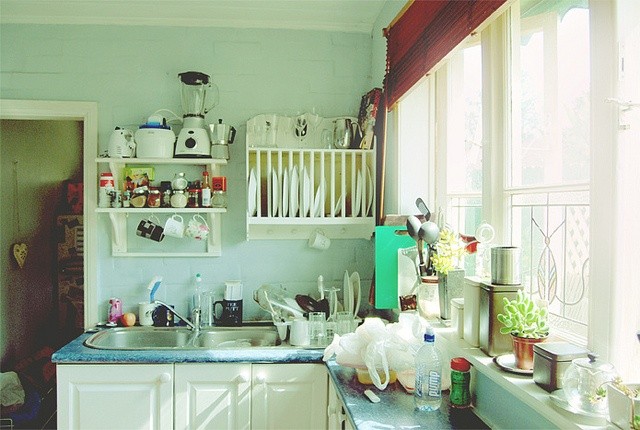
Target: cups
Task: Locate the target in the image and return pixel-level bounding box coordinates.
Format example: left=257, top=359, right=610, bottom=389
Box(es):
left=309, top=312, right=327, bottom=347
left=185, top=213, right=210, bottom=241
left=212, top=300, right=243, bottom=326
left=139, top=302, right=155, bottom=326
left=320, top=129, right=334, bottom=149
left=111, top=191, right=122, bottom=208
left=224, top=280, right=243, bottom=300
left=163, top=215, right=185, bottom=239
left=151, top=304, right=174, bottom=327
left=248, top=112, right=278, bottom=148
left=290, top=316, right=310, bottom=346
left=135, top=215, right=165, bottom=243
left=309, top=228, right=331, bottom=250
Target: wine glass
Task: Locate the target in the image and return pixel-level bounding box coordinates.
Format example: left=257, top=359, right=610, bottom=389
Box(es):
left=306, top=104, right=323, bottom=149
left=292, top=111, right=307, bottom=149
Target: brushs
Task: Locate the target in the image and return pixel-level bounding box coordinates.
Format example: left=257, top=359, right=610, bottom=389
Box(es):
left=147, top=278, right=162, bottom=302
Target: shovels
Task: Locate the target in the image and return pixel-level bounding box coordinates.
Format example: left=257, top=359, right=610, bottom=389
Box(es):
left=416, top=198, right=431, bottom=221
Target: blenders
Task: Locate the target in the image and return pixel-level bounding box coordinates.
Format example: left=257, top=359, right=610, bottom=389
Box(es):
left=174, top=71, right=219, bottom=158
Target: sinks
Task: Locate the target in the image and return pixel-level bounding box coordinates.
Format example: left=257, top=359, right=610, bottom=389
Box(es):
left=193, top=329, right=284, bottom=348
left=90, top=328, right=191, bottom=348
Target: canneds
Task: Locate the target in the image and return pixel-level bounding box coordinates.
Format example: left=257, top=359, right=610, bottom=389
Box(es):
left=147, top=188, right=161, bottom=208
left=187, top=189, right=203, bottom=208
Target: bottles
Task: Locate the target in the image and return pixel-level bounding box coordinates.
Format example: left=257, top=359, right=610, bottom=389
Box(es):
left=123, top=191, right=130, bottom=208
left=201, top=171, right=211, bottom=207
left=98, top=173, right=114, bottom=208
left=414, top=327, right=442, bottom=412
left=192, top=272, right=207, bottom=329
left=449, top=357, right=472, bottom=408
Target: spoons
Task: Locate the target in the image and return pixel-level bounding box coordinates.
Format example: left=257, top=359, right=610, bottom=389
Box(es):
left=419, top=221, right=439, bottom=275
left=406, top=215, right=426, bottom=275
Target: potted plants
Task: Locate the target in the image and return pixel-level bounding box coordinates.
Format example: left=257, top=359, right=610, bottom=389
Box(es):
left=498, top=288, right=552, bottom=371
left=589, top=375, right=639, bottom=430
left=428, top=226, right=466, bottom=320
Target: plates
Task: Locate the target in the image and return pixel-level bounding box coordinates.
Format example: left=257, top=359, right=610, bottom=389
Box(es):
left=247, top=168, right=256, bottom=217
left=290, top=166, right=299, bottom=217
left=281, top=168, right=289, bottom=216
left=313, top=181, right=322, bottom=217
left=271, top=168, right=279, bottom=217
left=302, top=166, right=311, bottom=217
left=343, top=269, right=354, bottom=315
left=295, top=295, right=329, bottom=320
left=335, top=193, right=342, bottom=216
left=350, top=272, right=361, bottom=319
left=550, top=389, right=610, bottom=416
left=366, top=166, right=374, bottom=216
left=354, top=167, right=362, bottom=218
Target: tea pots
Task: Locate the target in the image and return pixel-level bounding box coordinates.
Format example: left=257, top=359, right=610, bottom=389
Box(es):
left=106, top=126, right=136, bottom=158
left=208, top=118, right=236, bottom=145
left=561, top=353, right=615, bottom=414
left=332, top=118, right=363, bottom=149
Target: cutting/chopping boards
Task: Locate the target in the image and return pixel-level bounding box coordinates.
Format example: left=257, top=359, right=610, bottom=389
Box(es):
left=374, top=225, right=417, bottom=310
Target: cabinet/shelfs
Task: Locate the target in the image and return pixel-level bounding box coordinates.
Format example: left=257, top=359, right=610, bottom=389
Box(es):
left=175, top=364, right=328, bottom=429
left=329, top=376, right=352, bottom=429
left=96, top=158, right=227, bottom=259
left=246, top=111, right=376, bottom=240
left=56, top=364, right=175, bottom=429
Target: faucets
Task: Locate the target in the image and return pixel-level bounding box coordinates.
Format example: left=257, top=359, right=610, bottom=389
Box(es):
left=154, top=300, right=201, bottom=332
